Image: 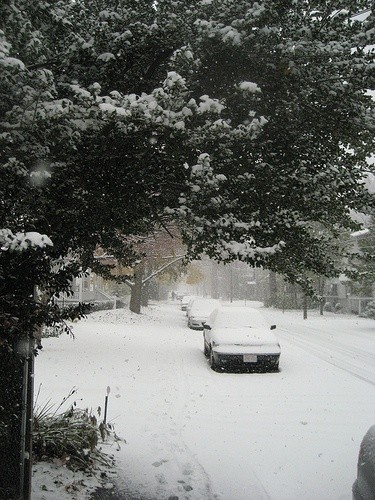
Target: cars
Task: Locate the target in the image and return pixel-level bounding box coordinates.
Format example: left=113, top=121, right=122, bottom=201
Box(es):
left=203, top=307, right=281, bottom=373
left=175, top=291, right=221, bottom=330
